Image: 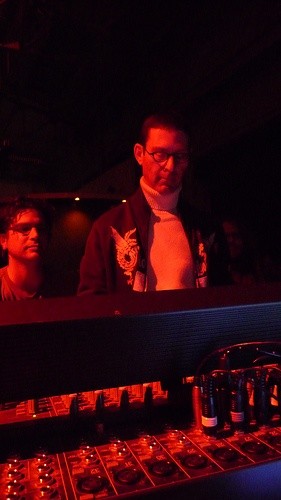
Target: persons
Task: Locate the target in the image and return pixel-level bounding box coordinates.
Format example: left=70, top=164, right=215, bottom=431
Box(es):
left=1, top=204, right=74, bottom=299
left=77, top=111, right=230, bottom=295
left=215, top=212, right=257, bottom=286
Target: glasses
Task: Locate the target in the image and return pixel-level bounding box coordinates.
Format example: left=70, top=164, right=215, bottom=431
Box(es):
left=8, top=223, right=44, bottom=232
left=144, top=147, right=190, bottom=162
